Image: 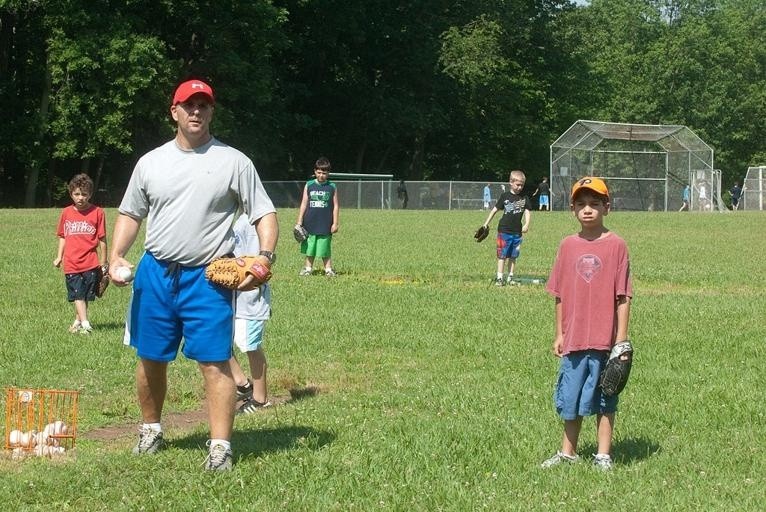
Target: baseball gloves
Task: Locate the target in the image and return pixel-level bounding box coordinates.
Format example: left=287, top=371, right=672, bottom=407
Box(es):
left=473, top=225, right=489, bottom=242
left=96, top=263, right=111, bottom=297
left=206, top=256, right=272, bottom=291
left=293, top=223, right=308, bottom=243
left=601, top=341, right=633, bottom=397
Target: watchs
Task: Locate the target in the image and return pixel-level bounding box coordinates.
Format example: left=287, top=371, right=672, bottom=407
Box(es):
left=259, top=251, right=276, bottom=264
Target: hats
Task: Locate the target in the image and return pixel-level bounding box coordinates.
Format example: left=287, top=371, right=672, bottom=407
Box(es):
left=173, top=80, right=215, bottom=105
left=572, top=177, right=609, bottom=199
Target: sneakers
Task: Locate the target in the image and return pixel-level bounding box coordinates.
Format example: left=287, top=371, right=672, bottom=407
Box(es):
left=238, top=398, right=271, bottom=413
left=134, top=425, right=163, bottom=453
left=205, top=443, right=233, bottom=471
left=69, top=324, right=94, bottom=334
left=592, top=453, right=611, bottom=469
left=325, top=270, right=335, bottom=277
left=495, top=276, right=516, bottom=286
left=541, top=450, right=578, bottom=467
left=236, top=379, right=252, bottom=401
left=300, top=268, right=310, bottom=277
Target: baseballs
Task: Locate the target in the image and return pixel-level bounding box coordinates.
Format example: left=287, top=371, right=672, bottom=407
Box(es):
left=116, top=267, right=132, bottom=282
left=7, top=421, right=69, bottom=458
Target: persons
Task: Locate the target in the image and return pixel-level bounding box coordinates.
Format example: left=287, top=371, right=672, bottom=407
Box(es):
left=484, top=182, right=492, bottom=210
left=483, top=170, right=531, bottom=286
left=540, top=176, right=632, bottom=477
left=678, top=181, right=743, bottom=212
left=109, top=80, right=278, bottom=475
left=294, top=158, right=339, bottom=277
left=226, top=200, right=272, bottom=416
left=53, top=173, right=107, bottom=336
left=533, top=177, right=556, bottom=211
left=499, top=184, right=506, bottom=195
left=397, top=179, right=408, bottom=209
